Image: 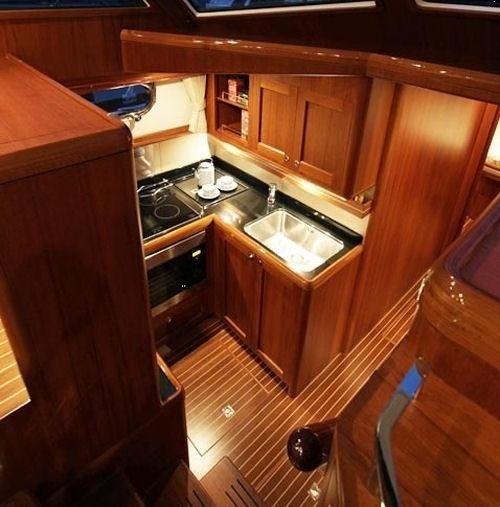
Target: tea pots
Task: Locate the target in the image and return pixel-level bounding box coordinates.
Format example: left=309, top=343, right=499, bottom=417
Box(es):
left=192, top=157, right=215, bottom=187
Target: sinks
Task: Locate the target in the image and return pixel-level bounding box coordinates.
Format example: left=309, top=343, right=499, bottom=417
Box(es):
left=243, top=209, right=345, bottom=274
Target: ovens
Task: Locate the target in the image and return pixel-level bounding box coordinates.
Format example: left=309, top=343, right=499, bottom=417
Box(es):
left=143, top=229, right=210, bottom=346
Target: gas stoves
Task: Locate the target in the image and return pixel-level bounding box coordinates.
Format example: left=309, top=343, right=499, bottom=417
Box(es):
left=138, top=179, right=201, bottom=241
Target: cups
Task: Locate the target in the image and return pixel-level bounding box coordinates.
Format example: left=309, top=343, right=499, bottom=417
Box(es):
left=219, top=175, right=234, bottom=188
left=201, top=184, right=217, bottom=196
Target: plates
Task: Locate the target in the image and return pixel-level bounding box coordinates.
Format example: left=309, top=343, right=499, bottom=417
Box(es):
left=216, top=181, right=237, bottom=191
left=198, top=188, right=220, bottom=199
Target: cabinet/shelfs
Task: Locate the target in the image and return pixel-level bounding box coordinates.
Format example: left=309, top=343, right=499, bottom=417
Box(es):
left=215, top=72, right=247, bottom=141
left=216, top=240, right=362, bottom=399
left=247, top=72, right=398, bottom=204
